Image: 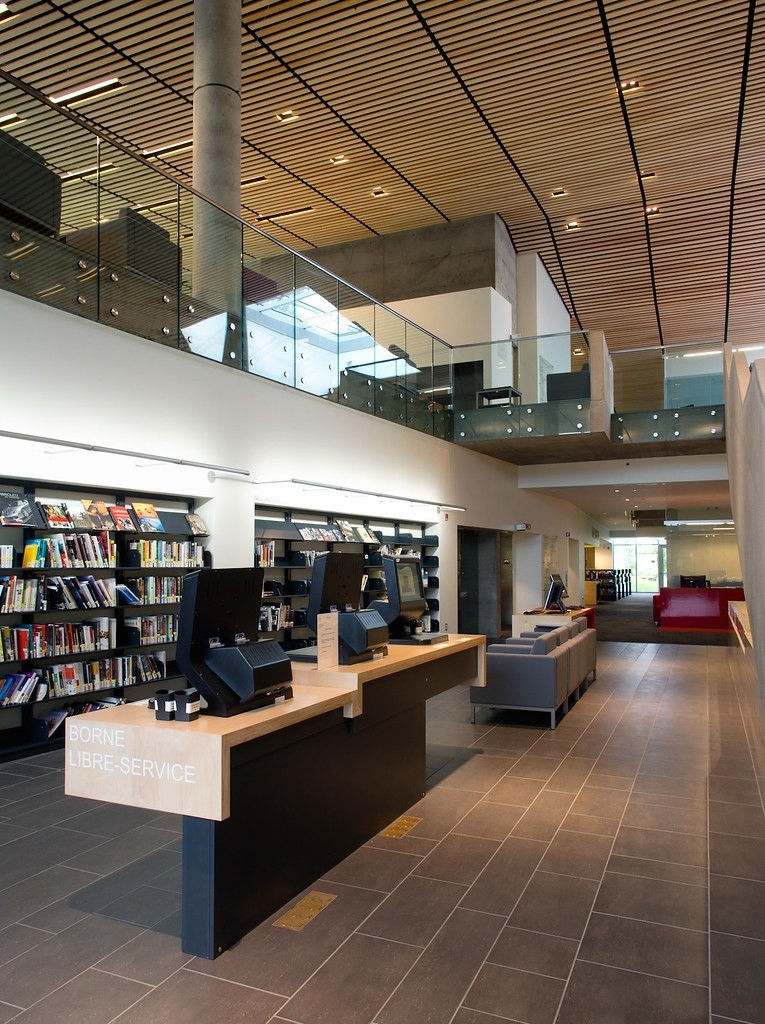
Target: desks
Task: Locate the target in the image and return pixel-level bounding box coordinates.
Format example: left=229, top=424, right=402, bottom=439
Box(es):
left=512, top=607, right=597, bottom=639
left=475, top=386, right=523, bottom=409
left=585, top=581, right=601, bottom=605
left=64, top=634, right=487, bottom=962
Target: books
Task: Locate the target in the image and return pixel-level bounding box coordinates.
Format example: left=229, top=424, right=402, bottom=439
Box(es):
left=0, top=650, right=123, bottom=706
left=256, top=540, right=275, bottom=567
left=300, top=550, right=330, bottom=566
left=122, top=650, right=166, bottom=686
left=421, top=615, right=431, bottom=632
left=41, top=697, right=127, bottom=737
left=185, top=512, right=209, bottom=535
left=127, top=539, right=204, bottom=568
left=361, top=567, right=389, bottom=600
left=262, top=578, right=312, bottom=598
left=0, top=492, right=165, bottom=533
left=0, top=617, right=116, bottom=662
left=297, top=637, right=317, bottom=649
left=422, top=570, right=428, bottom=588
left=117, top=576, right=184, bottom=605
left=298, top=519, right=380, bottom=544
left=0, top=573, right=116, bottom=613
left=375, top=544, right=421, bottom=563
left=258, top=602, right=294, bottom=632
left=0, top=531, right=117, bottom=570
left=125, top=614, right=179, bottom=645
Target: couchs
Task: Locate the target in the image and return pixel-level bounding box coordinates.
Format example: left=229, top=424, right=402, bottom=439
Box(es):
left=653, top=587, right=745, bottom=631
left=61, top=206, right=184, bottom=294
left=471, top=617, right=598, bottom=730
left=0, top=130, right=62, bottom=240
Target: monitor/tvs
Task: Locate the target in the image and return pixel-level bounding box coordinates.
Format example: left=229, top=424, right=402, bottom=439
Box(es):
left=550, top=574, right=570, bottom=612
left=544, top=580, right=567, bottom=614
left=396, top=562, right=422, bottom=600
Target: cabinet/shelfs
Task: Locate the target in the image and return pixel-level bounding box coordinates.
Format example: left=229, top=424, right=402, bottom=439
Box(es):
left=253, top=502, right=440, bottom=651
left=1, top=477, right=213, bottom=763
